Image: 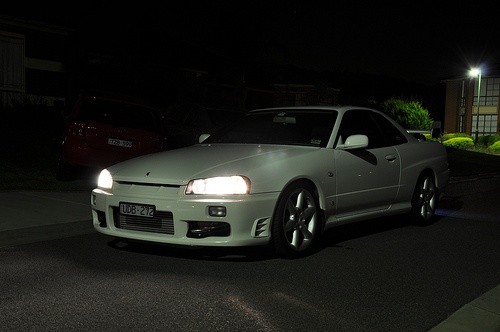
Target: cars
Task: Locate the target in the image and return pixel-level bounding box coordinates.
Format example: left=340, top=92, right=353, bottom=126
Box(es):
left=56, top=91, right=169, bottom=180
left=91, top=107, right=451, bottom=258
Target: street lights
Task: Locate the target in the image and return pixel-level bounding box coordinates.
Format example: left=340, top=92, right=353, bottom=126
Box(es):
left=466, top=67, right=485, bottom=147
